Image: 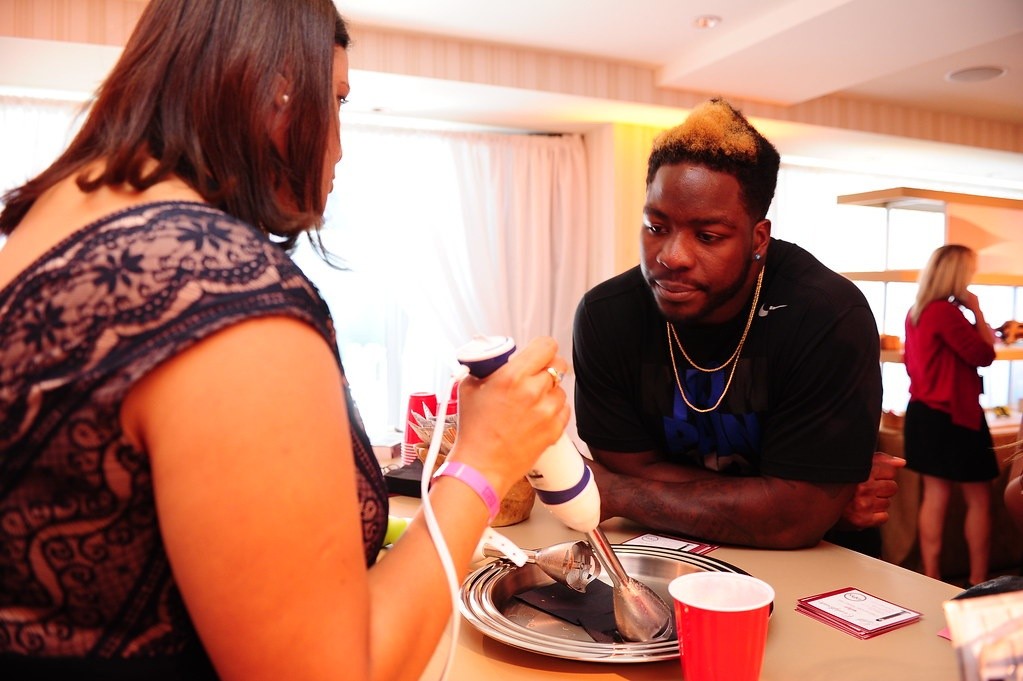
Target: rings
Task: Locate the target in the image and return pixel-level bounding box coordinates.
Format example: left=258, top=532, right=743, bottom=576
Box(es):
left=544, top=367, right=564, bottom=388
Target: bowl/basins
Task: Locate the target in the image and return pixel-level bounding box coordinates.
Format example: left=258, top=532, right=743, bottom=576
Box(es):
left=411, top=441, right=536, bottom=529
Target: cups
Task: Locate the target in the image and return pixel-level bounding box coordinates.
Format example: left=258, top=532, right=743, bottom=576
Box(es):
left=402, top=381, right=459, bottom=465
left=668, top=571, right=775, bottom=681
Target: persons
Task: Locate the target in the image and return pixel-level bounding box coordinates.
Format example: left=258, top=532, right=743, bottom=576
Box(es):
left=902, top=245, right=1001, bottom=588
left=0, top=0, right=573, bottom=681
left=572, top=96, right=883, bottom=551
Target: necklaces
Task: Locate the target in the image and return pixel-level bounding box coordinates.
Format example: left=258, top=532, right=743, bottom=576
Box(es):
left=666, top=264, right=766, bottom=413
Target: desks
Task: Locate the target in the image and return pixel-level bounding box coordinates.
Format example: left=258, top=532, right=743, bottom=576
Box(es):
left=379, top=495, right=966, bottom=681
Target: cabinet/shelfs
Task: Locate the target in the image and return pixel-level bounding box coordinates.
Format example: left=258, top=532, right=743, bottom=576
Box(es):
left=836, top=185, right=1023, bottom=364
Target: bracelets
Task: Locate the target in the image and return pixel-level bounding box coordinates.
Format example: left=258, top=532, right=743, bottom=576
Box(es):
left=430, top=461, right=500, bottom=525
